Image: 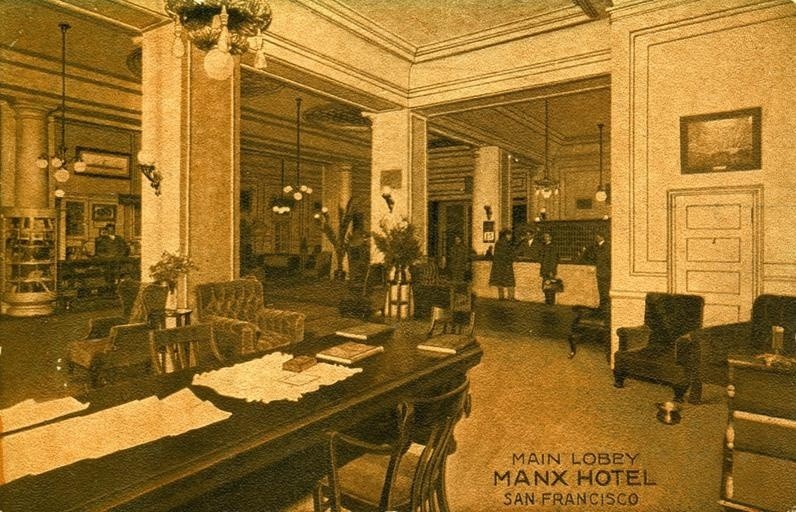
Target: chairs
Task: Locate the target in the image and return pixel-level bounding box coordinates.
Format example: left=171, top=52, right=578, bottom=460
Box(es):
left=568, top=300, right=610, bottom=365
left=312, top=375, right=473, bottom=512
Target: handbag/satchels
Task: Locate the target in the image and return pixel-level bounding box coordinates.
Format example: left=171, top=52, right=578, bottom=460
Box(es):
left=540, top=276, right=566, bottom=293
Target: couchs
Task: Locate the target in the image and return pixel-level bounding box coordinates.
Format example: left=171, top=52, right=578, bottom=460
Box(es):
left=674, top=291, right=796, bottom=405
left=338, top=263, right=388, bottom=321
left=62, top=278, right=170, bottom=391
left=194, top=279, right=306, bottom=358
left=409, top=259, right=473, bottom=334
left=613, top=291, right=705, bottom=403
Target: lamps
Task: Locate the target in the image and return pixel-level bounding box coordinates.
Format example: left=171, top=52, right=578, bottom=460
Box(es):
left=380, top=182, right=395, bottom=213
left=533, top=98, right=567, bottom=200
left=483, top=200, right=492, bottom=219
left=281, top=96, right=315, bottom=203
left=269, top=156, right=296, bottom=217
left=594, top=121, right=608, bottom=203
left=36, top=20, right=87, bottom=183
left=135, top=149, right=164, bottom=198
left=165, top=0, right=272, bottom=81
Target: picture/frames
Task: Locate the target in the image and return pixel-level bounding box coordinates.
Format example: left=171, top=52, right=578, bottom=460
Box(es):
left=677, top=106, right=764, bottom=177
left=92, top=204, right=118, bottom=222
left=75, top=145, right=132, bottom=180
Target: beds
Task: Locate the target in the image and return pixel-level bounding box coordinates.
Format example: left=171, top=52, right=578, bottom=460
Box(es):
left=151, top=307, right=191, bottom=374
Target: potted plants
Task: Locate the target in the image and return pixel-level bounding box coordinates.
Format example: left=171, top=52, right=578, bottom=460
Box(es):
left=146, top=249, right=188, bottom=375
left=370, top=212, right=425, bottom=283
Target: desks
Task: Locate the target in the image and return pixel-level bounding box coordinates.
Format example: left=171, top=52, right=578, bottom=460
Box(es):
left=2, top=314, right=485, bottom=511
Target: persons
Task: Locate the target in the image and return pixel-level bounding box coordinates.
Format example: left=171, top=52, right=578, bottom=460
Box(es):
left=514, top=230, right=540, bottom=261
left=594, top=232, right=611, bottom=306
left=486, top=246, right=493, bottom=255
left=447, top=235, right=471, bottom=282
left=540, top=230, right=558, bottom=313
left=489, top=230, right=520, bottom=303
left=96, top=222, right=129, bottom=299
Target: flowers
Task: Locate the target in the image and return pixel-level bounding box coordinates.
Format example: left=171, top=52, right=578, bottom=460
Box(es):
left=148, top=249, right=202, bottom=296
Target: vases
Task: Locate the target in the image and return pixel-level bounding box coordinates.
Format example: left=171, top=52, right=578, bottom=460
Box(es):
left=164, top=287, right=178, bottom=311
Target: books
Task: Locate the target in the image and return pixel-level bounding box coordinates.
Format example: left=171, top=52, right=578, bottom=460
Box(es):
left=335, top=320, right=391, bottom=341
left=315, top=341, right=385, bottom=364
left=417, top=334, right=477, bottom=354
